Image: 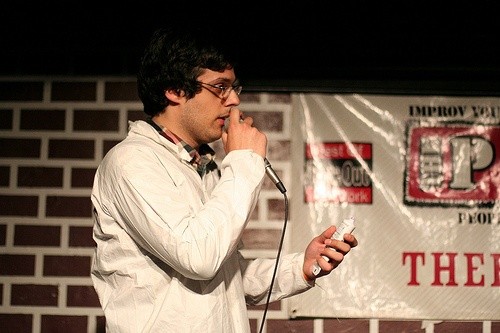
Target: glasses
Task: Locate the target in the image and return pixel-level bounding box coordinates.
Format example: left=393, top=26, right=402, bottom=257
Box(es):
left=196, top=81, right=243, bottom=99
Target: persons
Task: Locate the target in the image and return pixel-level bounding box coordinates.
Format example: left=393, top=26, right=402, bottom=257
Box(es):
left=90, top=33, right=359, bottom=333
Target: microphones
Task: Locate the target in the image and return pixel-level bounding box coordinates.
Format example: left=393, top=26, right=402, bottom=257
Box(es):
left=224, top=117, right=287, bottom=194
